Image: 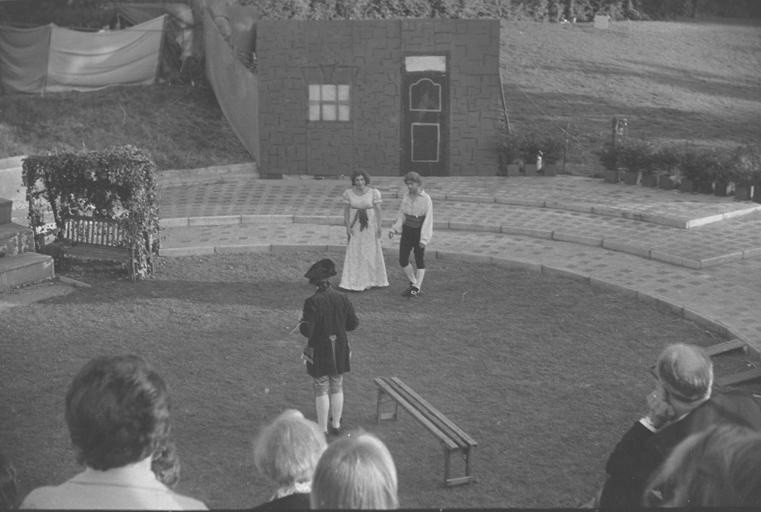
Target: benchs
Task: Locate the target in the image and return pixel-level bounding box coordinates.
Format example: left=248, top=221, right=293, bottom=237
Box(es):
left=373, top=377, right=479, bottom=488
left=35, top=212, right=151, bottom=282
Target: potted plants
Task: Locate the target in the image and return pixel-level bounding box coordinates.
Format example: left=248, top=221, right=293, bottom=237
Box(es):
left=493, top=130, right=522, bottom=176
left=537, top=135, right=565, bottom=176
left=596, top=135, right=760, bottom=203
left=517, top=131, right=543, bottom=176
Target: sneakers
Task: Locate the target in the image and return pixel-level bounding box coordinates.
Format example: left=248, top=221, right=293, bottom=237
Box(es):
left=410, top=285, right=418, bottom=295
left=401, top=288, right=411, bottom=296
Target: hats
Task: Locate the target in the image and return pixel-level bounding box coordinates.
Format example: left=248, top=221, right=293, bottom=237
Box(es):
left=304, top=259, right=337, bottom=280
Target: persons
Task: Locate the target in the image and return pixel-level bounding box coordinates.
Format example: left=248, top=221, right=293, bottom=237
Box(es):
left=339, top=169, right=391, bottom=291
left=643, top=391, right=761, bottom=511
left=299, top=258, right=360, bottom=436
left=250, top=408, right=327, bottom=512
left=312, top=427, right=399, bottom=512
left=600, top=342, right=713, bottom=512
left=0, top=455, right=22, bottom=511
left=17, top=354, right=209, bottom=511
left=150, top=443, right=180, bottom=491
left=392, top=172, right=435, bottom=295
